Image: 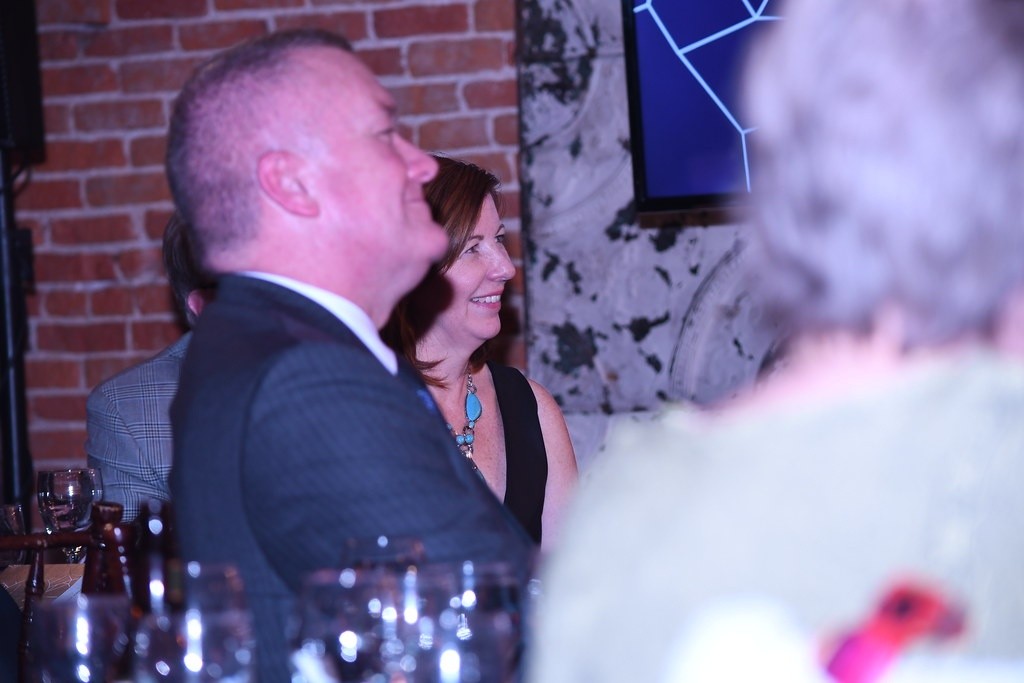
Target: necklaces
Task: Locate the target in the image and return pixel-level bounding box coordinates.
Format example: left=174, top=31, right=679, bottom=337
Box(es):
left=444, top=366, right=482, bottom=460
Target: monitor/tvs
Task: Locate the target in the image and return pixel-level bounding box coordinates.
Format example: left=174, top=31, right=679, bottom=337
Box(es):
left=621, top=0, right=781, bottom=209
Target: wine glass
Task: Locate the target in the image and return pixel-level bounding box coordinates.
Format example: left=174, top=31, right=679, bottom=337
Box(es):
left=38, top=466, right=102, bottom=564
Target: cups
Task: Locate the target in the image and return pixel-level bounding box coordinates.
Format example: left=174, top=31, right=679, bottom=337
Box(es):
left=31, top=533, right=521, bottom=683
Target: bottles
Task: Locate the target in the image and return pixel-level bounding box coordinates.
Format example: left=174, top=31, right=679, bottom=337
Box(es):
left=65, top=502, right=134, bottom=683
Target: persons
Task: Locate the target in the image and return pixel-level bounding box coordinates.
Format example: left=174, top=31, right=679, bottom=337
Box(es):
left=86, top=0, right=1024, bottom=683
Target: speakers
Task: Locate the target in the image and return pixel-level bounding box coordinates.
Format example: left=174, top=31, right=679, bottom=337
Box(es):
left=0, top=0, right=46, bottom=156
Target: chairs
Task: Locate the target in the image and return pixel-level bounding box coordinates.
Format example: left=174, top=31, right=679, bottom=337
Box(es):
left=0, top=500, right=186, bottom=682
